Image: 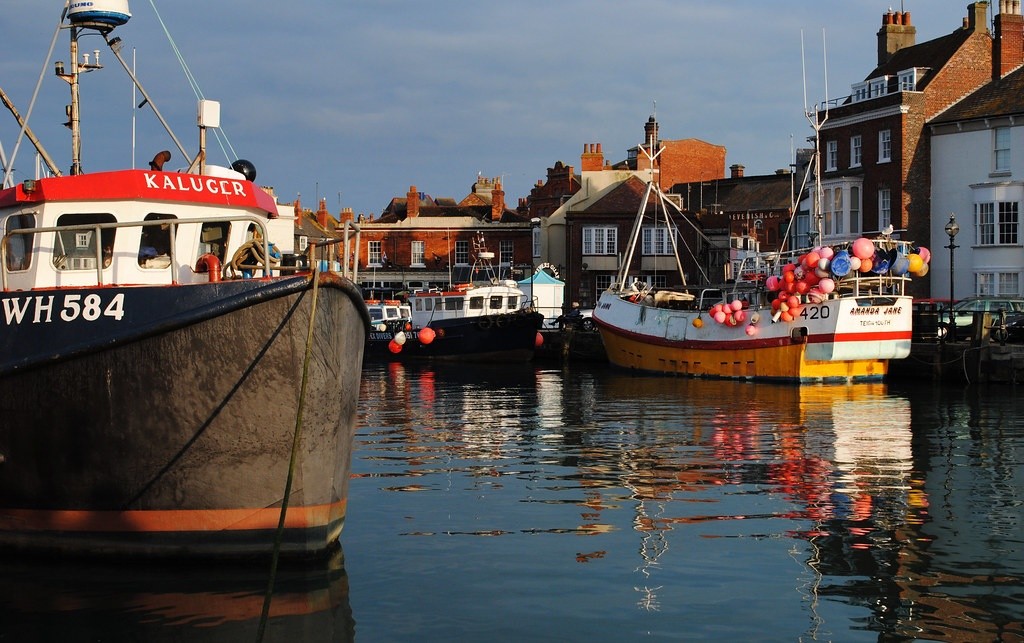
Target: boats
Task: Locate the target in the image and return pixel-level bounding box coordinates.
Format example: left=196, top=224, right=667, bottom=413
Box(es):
left=366, top=227, right=544, bottom=360
left=1, top=0, right=374, bottom=575
left=590, top=100, right=914, bottom=388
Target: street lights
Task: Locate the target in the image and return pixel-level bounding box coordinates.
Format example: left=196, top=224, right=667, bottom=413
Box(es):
left=528, top=217, right=540, bottom=311
left=944, top=212, right=961, bottom=343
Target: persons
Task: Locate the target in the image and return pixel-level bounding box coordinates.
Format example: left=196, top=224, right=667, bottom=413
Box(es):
left=549, top=302, right=579, bottom=329
left=138, top=232, right=157, bottom=268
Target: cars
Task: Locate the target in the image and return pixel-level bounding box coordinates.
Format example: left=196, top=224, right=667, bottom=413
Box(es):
left=579, top=308, right=598, bottom=332
left=912, top=295, right=1024, bottom=345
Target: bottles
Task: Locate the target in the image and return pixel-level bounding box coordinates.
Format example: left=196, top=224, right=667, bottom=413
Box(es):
left=771, top=309, right=782, bottom=323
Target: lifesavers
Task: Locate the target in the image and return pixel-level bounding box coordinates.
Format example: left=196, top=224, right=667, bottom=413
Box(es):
left=364, top=299, right=382, bottom=305
left=741, top=273, right=768, bottom=281
left=382, top=299, right=401, bottom=306
left=451, top=282, right=473, bottom=292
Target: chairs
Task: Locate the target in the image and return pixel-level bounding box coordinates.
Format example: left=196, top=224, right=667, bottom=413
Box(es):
left=562, top=315, right=588, bottom=333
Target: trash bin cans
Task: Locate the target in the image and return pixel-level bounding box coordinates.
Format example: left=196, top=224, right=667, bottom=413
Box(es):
left=912, top=302, right=938, bottom=343
